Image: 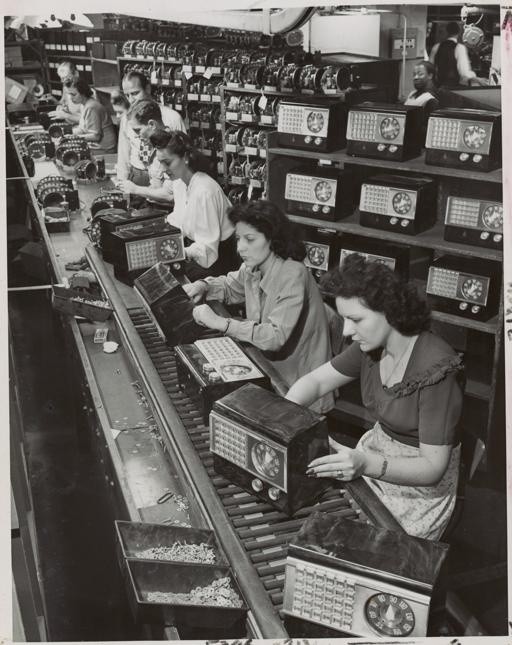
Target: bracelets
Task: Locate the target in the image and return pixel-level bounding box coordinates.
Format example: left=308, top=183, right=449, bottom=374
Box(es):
left=375, top=453, right=390, bottom=482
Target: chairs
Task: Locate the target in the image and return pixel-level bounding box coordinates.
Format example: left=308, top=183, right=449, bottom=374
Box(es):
left=422, top=428, right=508, bottom=635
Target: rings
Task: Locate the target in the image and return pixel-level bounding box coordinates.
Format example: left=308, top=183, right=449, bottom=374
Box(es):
left=336, top=469, right=344, bottom=480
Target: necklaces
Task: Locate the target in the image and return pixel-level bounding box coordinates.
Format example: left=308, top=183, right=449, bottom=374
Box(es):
left=381, top=337, right=411, bottom=390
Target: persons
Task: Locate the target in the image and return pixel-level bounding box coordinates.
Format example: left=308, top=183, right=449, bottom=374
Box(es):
left=401, top=59, right=452, bottom=116
left=116, top=69, right=187, bottom=212
left=281, top=251, right=468, bottom=544
left=47, top=60, right=98, bottom=128
left=63, top=75, right=118, bottom=155
left=427, top=20, right=487, bottom=90
left=149, top=128, right=244, bottom=282
left=181, top=197, right=341, bottom=420
left=115, top=99, right=175, bottom=214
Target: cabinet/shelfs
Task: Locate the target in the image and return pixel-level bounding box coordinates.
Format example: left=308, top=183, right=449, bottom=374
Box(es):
left=223, top=82, right=335, bottom=196
left=182, top=56, right=229, bottom=154
left=118, top=36, right=182, bottom=131
left=266, top=132, right=503, bottom=456
